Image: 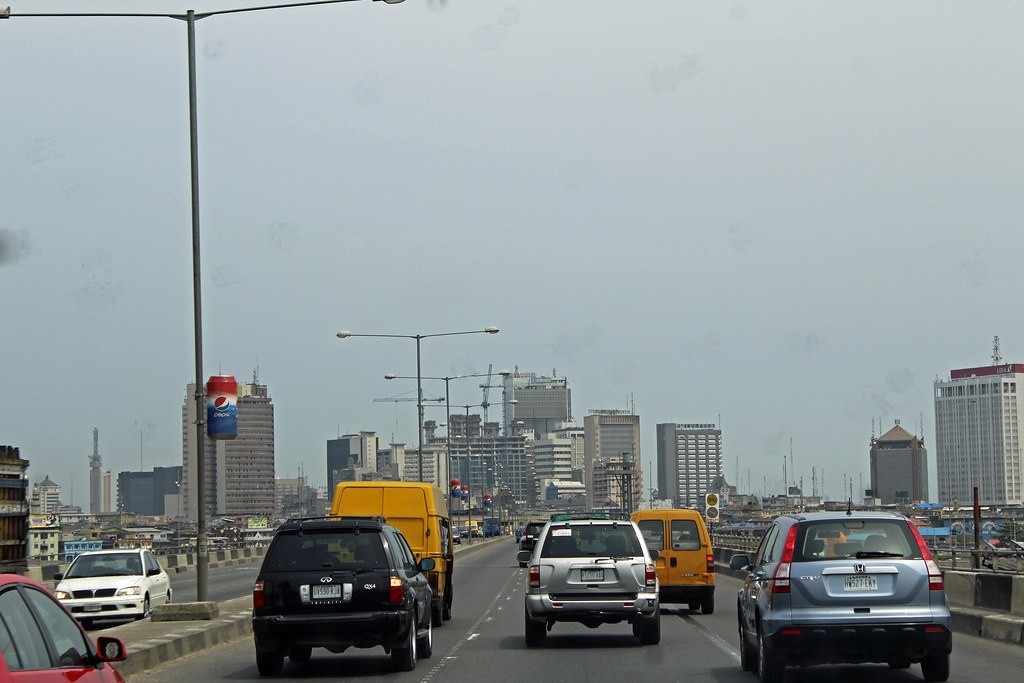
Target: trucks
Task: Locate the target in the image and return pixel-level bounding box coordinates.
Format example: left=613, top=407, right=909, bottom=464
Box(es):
left=483, top=516, right=501, bottom=538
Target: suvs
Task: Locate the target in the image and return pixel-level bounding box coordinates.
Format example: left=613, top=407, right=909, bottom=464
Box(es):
left=518, top=519, right=551, bottom=567
left=729, top=510, right=953, bottom=683
left=515, top=511, right=662, bottom=645
left=251, top=516, right=435, bottom=675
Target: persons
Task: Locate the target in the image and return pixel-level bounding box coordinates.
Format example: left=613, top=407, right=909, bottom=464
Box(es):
left=46, top=514, right=57, bottom=526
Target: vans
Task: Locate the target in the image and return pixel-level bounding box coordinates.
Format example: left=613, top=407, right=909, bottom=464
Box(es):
left=627, top=511, right=714, bottom=613
left=466, top=521, right=478, bottom=538
left=327, top=481, right=455, bottom=626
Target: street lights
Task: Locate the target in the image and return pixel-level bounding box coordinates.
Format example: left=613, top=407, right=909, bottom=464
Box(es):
left=0, top=0, right=405, bottom=602
left=336, top=327, right=499, bottom=482
left=467, top=445, right=541, bottom=536
left=441, top=421, right=524, bottom=539
left=416, top=400, right=518, bottom=544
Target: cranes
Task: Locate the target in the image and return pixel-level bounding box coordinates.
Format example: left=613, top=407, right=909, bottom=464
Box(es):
left=482, top=363, right=492, bottom=424
left=372, top=388, right=445, bottom=445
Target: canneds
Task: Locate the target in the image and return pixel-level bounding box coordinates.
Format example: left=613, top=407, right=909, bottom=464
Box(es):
left=206, top=375, right=237, bottom=440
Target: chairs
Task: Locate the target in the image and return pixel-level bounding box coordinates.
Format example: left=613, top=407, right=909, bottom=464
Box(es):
left=834, top=542, right=862, bottom=557
left=605, top=535, right=626, bottom=557
left=554, top=535, right=578, bottom=556
left=813, top=540, right=825, bottom=558
left=353, top=545, right=377, bottom=568
left=865, top=535, right=895, bottom=553
left=93, top=566, right=105, bottom=575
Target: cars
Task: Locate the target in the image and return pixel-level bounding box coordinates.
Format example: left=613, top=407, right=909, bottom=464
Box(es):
left=452, top=526, right=461, bottom=545
left=0, top=573, right=127, bottom=683
left=516, top=527, right=525, bottom=543
left=52, top=548, right=173, bottom=627
left=458, top=526, right=468, bottom=539
